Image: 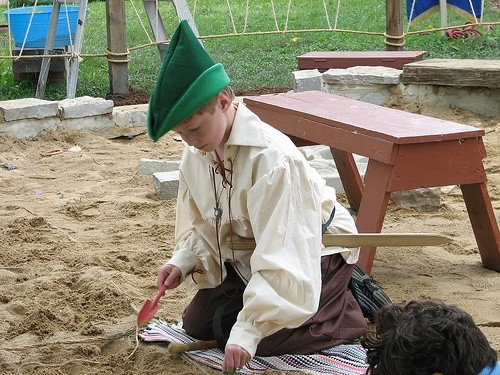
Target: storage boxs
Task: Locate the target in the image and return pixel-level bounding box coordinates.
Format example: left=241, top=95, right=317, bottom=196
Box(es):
left=1, top=5, right=89, bottom=47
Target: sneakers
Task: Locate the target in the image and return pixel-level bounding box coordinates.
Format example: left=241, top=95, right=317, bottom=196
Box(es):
left=348, top=262, right=394, bottom=324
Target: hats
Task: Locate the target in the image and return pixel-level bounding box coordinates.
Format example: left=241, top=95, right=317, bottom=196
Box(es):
left=146, top=20, right=232, bottom=143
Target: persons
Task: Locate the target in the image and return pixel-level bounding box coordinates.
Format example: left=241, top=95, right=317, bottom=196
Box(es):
left=359, top=299, right=500, bottom=375
left=147, top=20, right=393, bottom=373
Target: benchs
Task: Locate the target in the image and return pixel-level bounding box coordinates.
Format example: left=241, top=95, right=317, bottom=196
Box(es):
left=295, top=49, right=427, bottom=68
left=242, top=90, right=500, bottom=274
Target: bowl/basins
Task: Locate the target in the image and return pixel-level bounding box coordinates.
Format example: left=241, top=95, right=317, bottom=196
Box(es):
left=4, top=6, right=89, bottom=47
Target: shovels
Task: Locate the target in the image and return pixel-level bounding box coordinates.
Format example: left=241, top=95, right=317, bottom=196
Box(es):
left=136, top=283, right=167, bottom=327
